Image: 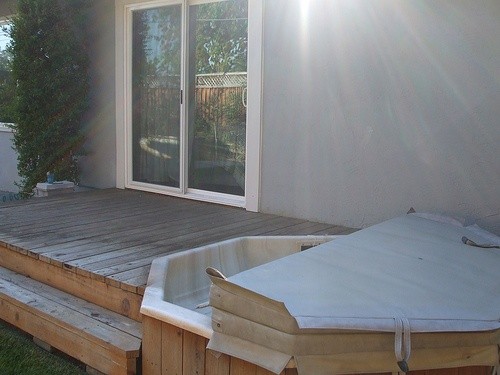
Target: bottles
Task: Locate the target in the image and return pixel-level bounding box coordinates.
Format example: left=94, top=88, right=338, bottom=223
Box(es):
left=47, top=170, right=54, bottom=184
